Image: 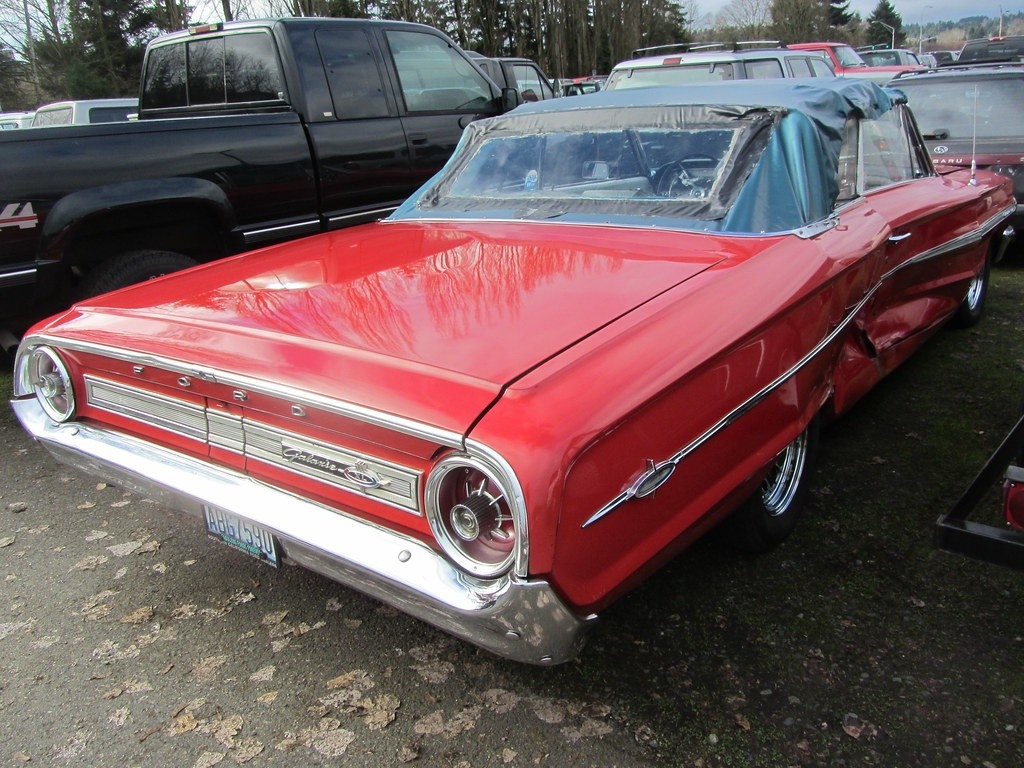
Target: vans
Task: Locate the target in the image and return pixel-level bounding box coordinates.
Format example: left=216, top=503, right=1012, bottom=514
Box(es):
left=31, top=95, right=140, bottom=127
left=0, top=111, right=37, bottom=131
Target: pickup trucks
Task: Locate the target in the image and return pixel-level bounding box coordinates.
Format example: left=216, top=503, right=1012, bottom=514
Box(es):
left=0, top=18, right=521, bottom=342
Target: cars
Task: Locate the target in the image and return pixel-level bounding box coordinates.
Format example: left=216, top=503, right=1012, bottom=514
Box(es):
left=7, top=75, right=1018, bottom=667
left=395, top=36, right=1024, bottom=268
